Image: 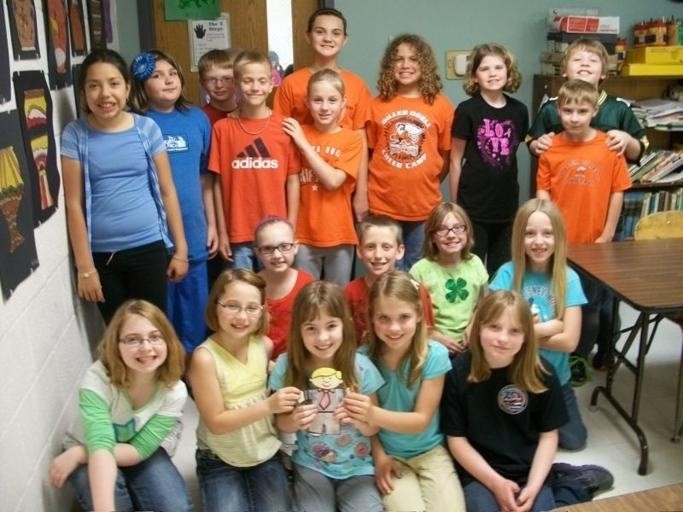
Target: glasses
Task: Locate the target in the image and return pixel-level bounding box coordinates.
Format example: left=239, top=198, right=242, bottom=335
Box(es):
left=431, top=224, right=467, bottom=236
left=215, top=300, right=264, bottom=315
left=117, top=334, right=163, bottom=344
left=256, top=243, right=295, bottom=253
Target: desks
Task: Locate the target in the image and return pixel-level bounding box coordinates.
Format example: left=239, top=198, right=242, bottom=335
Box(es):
left=566, top=237, right=682, bottom=474
left=539, top=482, right=683, bottom=512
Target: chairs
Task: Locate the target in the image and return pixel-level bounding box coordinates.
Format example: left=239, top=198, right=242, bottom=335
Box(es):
left=633, top=210, right=682, bottom=443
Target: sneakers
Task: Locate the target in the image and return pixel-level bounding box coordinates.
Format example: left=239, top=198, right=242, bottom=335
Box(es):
left=552, top=463, right=613, bottom=492
left=569, top=344, right=614, bottom=387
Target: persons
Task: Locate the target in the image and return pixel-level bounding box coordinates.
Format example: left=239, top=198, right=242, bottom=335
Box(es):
left=50, top=7, right=650, bottom=512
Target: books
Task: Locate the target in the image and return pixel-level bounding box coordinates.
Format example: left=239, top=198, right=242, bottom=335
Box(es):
left=613, top=95, right=682, bottom=243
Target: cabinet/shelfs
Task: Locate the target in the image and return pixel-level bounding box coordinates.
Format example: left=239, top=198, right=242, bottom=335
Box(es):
left=530, top=74, right=683, bottom=242
